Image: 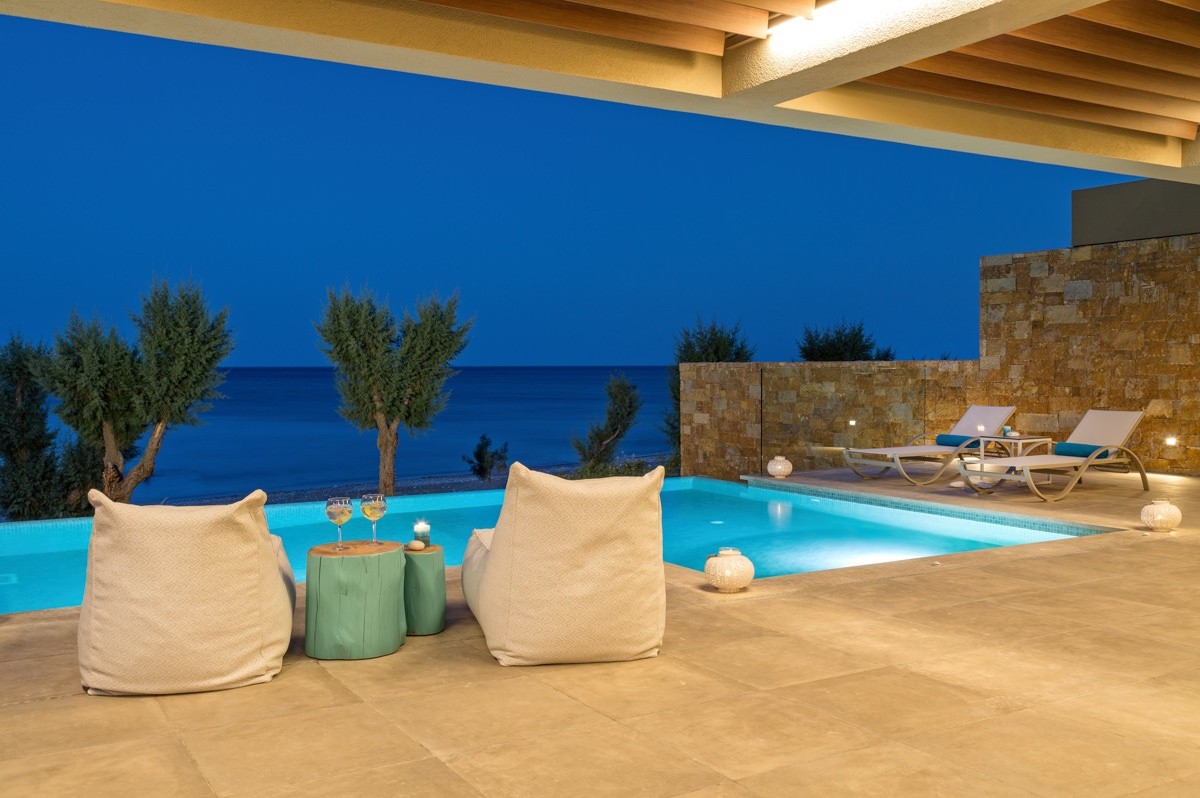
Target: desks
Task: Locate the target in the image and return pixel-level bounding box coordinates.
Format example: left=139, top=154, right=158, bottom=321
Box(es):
left=402, top=542, right=448, bottom=637
left=302, top=539, right=407, bottom=664
left=976, top=434, right=1053, bottom=488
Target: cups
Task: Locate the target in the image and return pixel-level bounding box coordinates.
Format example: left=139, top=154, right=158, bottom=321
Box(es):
left=414, top=525, right=431, bottom=547
left=1003, top=426, right=1011, bottom=436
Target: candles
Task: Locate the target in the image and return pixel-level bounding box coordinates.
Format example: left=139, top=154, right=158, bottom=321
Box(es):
left=1002, top=425, right=1012, bottom=436
left=412, top=517, right=431, bottom=549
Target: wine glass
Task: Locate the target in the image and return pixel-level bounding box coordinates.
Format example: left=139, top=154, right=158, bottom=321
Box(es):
left=325, top=497, right=353, bottom=552
left=359, top=494, right=387, bottom=546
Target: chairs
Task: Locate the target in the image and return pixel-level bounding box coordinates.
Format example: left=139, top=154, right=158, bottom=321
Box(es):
left=956, top=408, right=1152, bottom=504
left=73, top=485, right=297, bottom=697
left=459, top=458, right=667, bottom=668
left=842, top=404, right=1017, bottom=488
